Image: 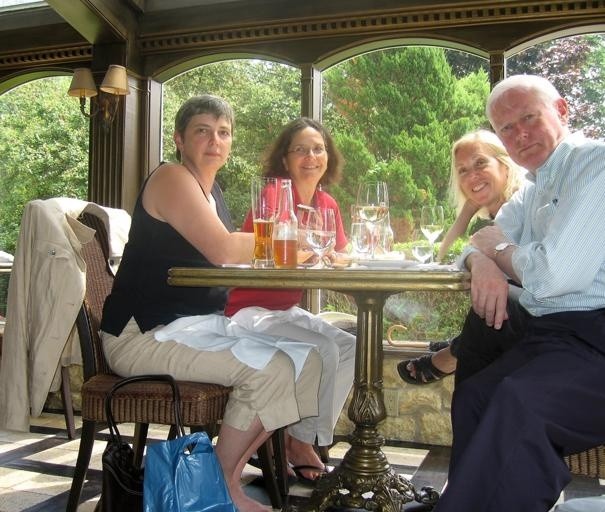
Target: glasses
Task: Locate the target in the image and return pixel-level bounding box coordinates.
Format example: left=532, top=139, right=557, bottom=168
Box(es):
left=286, top=144, right=325, bottom=156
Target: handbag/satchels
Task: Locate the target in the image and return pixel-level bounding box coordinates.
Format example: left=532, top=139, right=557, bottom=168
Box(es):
left=95, top=374, right=192, bottom=512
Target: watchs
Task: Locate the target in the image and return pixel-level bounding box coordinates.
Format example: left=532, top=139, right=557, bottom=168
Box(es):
left=493, top=241, right=521, bottom=262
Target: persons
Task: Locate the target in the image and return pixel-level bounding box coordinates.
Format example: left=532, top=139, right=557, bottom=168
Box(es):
left=99, top=91, right=325, bottom=512
left=428, top=70, right=605, bottom=512
left=398, top=128, right=540, bottom=387
left=223, top=115, right=359, bottom=485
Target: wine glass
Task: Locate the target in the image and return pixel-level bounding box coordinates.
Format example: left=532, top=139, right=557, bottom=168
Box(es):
left=304, top=178, right=448, bottom=269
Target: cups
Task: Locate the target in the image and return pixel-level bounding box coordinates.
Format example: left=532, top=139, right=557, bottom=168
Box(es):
left=247, top=174, right=280, bottom=272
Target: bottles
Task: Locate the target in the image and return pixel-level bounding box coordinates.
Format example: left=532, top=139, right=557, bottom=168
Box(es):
left=273, top=177, right=298, bottom=269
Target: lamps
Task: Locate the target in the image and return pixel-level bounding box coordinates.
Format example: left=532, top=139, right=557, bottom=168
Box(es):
left=66, top=65, right=131, bottom=125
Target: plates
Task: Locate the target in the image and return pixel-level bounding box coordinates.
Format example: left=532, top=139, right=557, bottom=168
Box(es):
left=353, top=255, right=421, bottom=270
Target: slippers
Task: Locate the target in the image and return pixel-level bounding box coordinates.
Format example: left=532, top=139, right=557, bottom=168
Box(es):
left=249, top=456, right=298, bottom=481
left=295, top=458, right=328, bottom=485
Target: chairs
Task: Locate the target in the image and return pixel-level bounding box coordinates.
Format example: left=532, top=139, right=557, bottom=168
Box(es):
left=29, top=200, right=284, bottom=512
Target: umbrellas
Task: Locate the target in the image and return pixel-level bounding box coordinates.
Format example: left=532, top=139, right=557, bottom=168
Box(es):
left=386, top=324, right=459, bottom=351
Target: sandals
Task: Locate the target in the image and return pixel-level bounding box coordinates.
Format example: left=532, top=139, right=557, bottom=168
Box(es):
left=398, top=354, right=457, bottom=386
